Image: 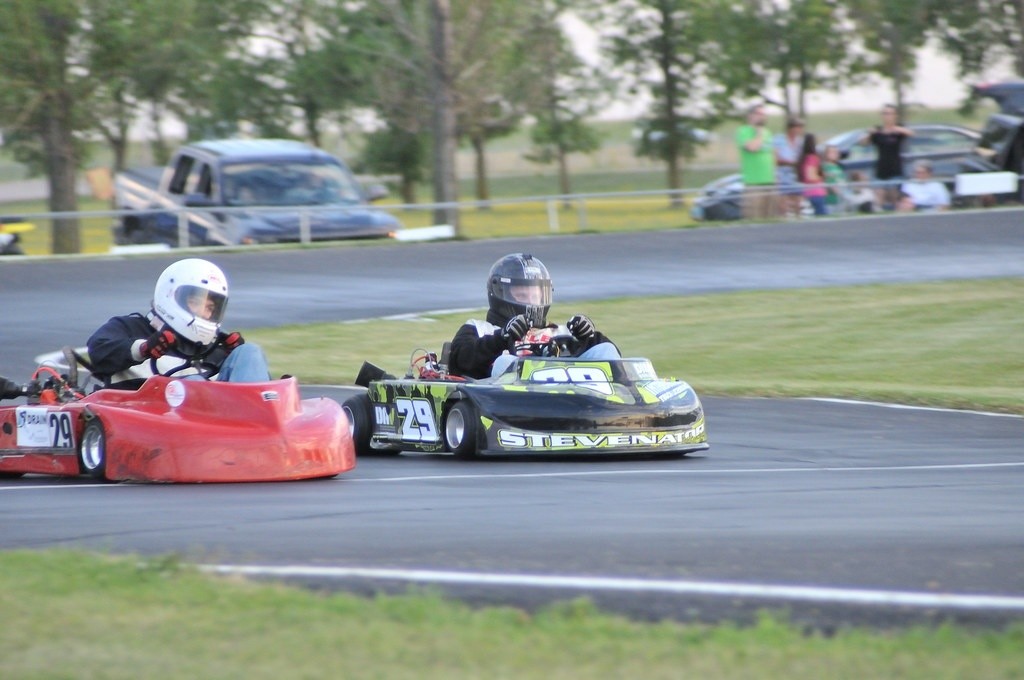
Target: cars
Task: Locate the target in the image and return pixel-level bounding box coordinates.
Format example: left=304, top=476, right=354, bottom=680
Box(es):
left=686, top=79, right=1024, bottom=223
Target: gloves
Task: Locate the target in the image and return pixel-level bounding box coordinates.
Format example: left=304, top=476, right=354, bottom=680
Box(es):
left=567, top=315, right=596, bottom=341
left=139, top=331, right=175, bottom=359
left=224, top=332, right=244, bottom=354
left=501, top=314, right=533, bottom=340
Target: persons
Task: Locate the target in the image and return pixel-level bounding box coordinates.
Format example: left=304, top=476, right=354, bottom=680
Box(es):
left=738, top=106, right=951, bottom=217
left=0, top=374, right=70, bottom=399
left=449, top=252, right=621, bottom=381
left=86, top=258, right=269, bottom=391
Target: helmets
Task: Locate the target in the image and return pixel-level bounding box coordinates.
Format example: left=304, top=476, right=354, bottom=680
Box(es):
left=487, top=253, right=553, bottom=327
left=154, top=258, right=228, bottom=346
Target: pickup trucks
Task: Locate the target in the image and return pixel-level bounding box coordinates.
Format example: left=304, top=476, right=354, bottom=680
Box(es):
left=115, top=139, right=400, bottom=248
